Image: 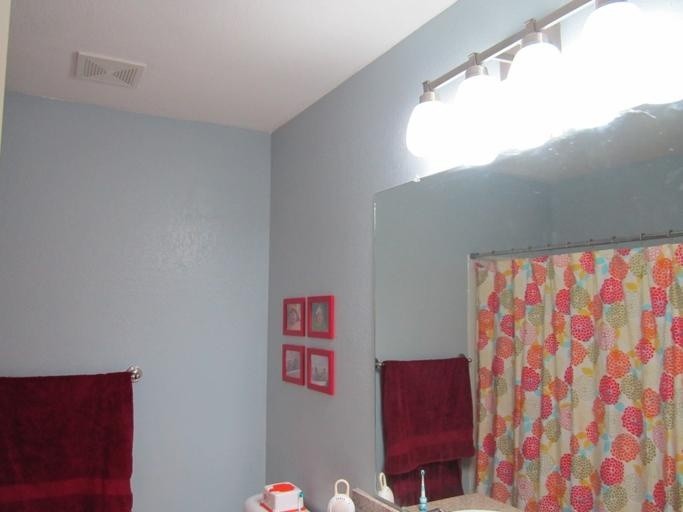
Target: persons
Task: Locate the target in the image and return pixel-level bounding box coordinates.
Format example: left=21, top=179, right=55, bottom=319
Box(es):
left=312, top=306, right=329, bottom=332
left=289, top=307, right=301, bottom=330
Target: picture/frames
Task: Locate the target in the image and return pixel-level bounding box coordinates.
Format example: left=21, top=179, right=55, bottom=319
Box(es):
left=280, top=296, right=306, bottom=338
left=280, top=343, right=305, bottom=386
left=305, top=293, right=336, bottom=340
left=304, top=346, right=335, bottom=395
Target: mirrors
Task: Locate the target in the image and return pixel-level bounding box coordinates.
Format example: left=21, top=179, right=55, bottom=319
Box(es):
left=371, top=98, right=682, bottom=512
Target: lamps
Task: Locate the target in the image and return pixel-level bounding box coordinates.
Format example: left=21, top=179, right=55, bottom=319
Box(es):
left=405, top=0, right=683, bottom=178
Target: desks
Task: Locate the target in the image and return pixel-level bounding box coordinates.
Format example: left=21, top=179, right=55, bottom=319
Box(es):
left=403, top=491, right=522, bottom=512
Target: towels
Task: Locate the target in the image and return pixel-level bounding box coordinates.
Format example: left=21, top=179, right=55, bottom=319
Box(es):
left=377, top=351, right=478, bottom=507
left=0, top=369, right=134, bottom=512
